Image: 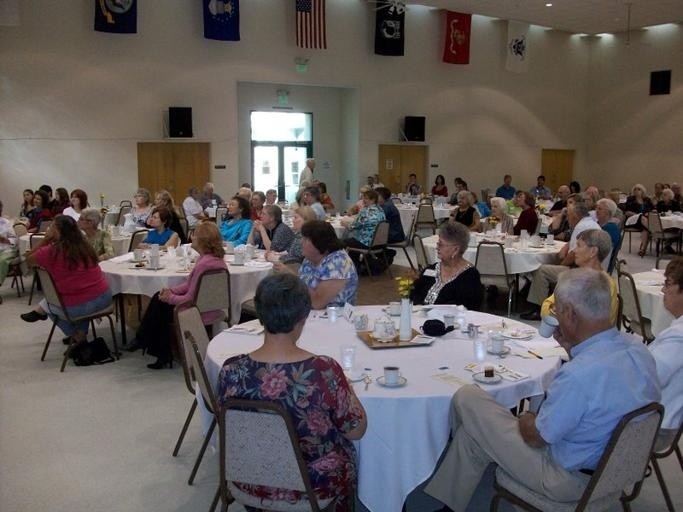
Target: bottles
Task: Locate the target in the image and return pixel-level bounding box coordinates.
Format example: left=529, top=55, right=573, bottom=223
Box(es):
left=399, top=298, right=412, bottom=341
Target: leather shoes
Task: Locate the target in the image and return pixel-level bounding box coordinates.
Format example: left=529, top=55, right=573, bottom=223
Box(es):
left=20, top=310, right=48, bottom=322
left=520, top=311, right=541, bottom=321
left=387, top=249, right=396, bottom=264
left=63, top=336, right=86, bottom=345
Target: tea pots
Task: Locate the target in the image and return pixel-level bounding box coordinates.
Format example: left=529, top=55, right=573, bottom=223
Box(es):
left=368, top=313, right=400, bottom=342
left=122, top=213, right=138, bottom=232
left=419, top=319, right=454, bottom=336
left=150, top=244, right=160, bottom=266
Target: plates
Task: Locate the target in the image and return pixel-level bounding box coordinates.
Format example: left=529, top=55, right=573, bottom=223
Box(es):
left=350, top=366, right=369, bottom=382
left=545, top=242, right=557, bottom=245
left=486, top=344, right=511, bottom=355
left=472, top=371, right=503, bottom=385
left=502, top=329, right=532, bottom=339
left=229, top=262, right=245, bottom=267
left=146, top=265, right=166, bottom=270
left=447, top=322, right=461, bottom=330
left=128, top=259, right=148, bottom=263
left=224, top=250, right=235, bottom=255
left=375, top=376, right=407, bottom=387
left=529, top=243, right=544, bottom=248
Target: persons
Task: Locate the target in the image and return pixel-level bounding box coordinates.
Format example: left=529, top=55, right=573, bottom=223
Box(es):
left=1, top=156, right=682, bottom=369
left=647, top=260, right=683, bottom=451
left=214, top=273, right=368, bottom=511
left=423, top=265, right=660, bottom=511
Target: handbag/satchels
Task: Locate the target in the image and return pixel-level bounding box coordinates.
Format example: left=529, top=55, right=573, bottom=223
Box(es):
left=72, top=337, right=115, bottom=366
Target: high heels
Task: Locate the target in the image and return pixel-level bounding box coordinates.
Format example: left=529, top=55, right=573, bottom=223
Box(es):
left=147, top=358, right=173, bottom=369
left=118, top=338, right=146, bottom=356
left=638, top=248, right=649, bottom=258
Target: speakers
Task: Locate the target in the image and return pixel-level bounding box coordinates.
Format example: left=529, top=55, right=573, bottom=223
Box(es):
left=405, top=116, right=425, bottom=141
left=169, top=106, right=192, bottom=137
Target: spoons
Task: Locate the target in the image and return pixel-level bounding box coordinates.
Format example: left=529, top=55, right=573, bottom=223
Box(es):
left=362, top=377, right=372, bottom=391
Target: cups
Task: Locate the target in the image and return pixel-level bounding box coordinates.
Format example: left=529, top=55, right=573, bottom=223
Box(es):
left=388, top=301, right=401, bottom=314
left=538, top=315, right=560, bottom=338
left=134, top=248, right=143, bottom=260
left=150, top=256, right=161, bottom=267
left=234, top=253, right=244, bottom=264
left=353, top=312, right=369, bottom=331
left=326, top=303, right=339, bottom=323
left=491, top=336, right=505, bottom=351
left=547, top=234, right=555, bottom=243
left=533, top=236, right=544, bottom=246
left=111, top=226, right=120, bottom=237
left=467, top=323, right=480, bottom=339
left=382, top=366, right=402, bottom=384
left=340, top=346, right=356, bottom=368
left=443, top=313, right=456, bottom=328
left=521, top=229, right=529, bottom=250
left=484, top=365, right=495, bottom=377
left=226, top=242, right=234, bottom=252
left=474, top=340, right=488, bottom=362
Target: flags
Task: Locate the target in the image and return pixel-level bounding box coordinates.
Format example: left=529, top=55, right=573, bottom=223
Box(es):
left=504, top=20, right=531, bottom=75
left=94, top=1, right=137, bottom=35
left=443, top=10, right=472, bottom=64
left=374, top=1, right=406, bottom=56
left=295, top=0, right=327, bottom=48
left=202, top=0, right=242, bottom=41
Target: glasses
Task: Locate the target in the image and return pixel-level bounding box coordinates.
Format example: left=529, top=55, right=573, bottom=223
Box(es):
left=665, top=279, right=678, bottom=287
left=437, top=241, right=459, bottom=247
